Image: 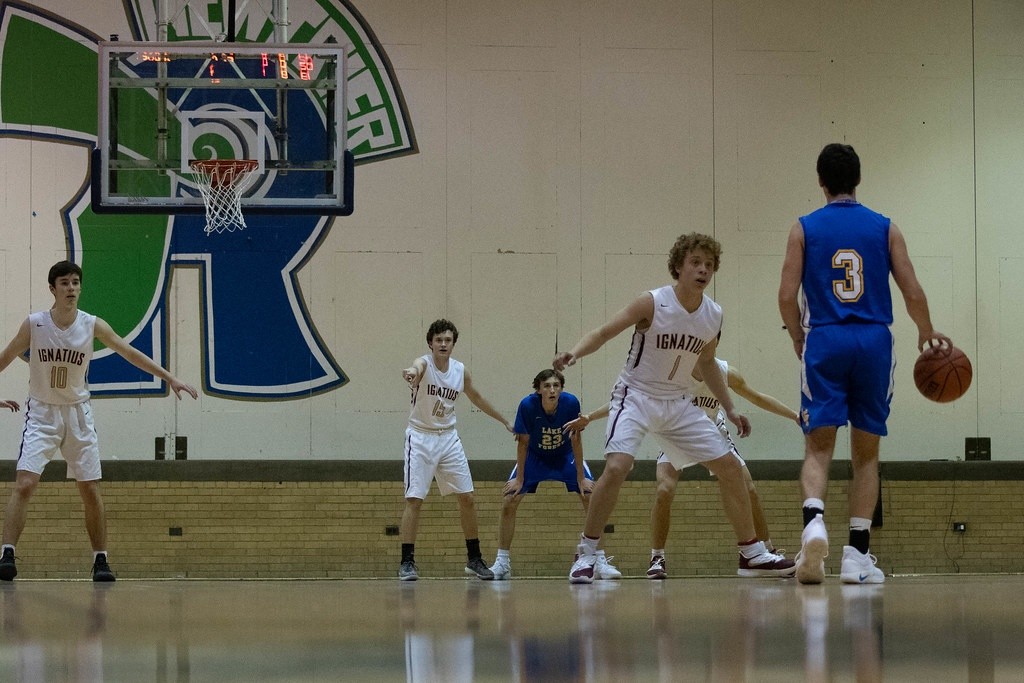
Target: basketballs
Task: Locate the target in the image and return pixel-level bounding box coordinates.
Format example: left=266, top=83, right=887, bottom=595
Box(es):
left=912, top=343, right=974, bottom=405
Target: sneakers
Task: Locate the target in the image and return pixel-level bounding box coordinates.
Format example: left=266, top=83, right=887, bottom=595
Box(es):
left=839, top=546, right=885, bottom=583
left=645, top=556, right=668, bottom=579
left=593, top=556, right=621, bottom=579
left=89, top=553, right=115, bottom=581
left=769, top=549, right=795, bottom=578
left=0, top=547, right=23, bottom=581
left=569, top=544, right=598, bottom=584
left=465, top=556, right=494, bottom=579
left=400, top=560, right=419, bottom=581
left=794, top=514, right=829, bottom=584
left=476, top=561, right=511, bottom=580
left=737, top=541, right=795, bottom=577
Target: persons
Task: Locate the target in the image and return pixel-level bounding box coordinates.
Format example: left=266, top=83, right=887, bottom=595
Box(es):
left=398, top=319, right=519, bottom=581
left=0, top=400, right=20, bottom=412
left=563, top=329, right=801, bottom=577
left=0, top=582, right=109, bottom=683
left=550, top=232, right=796, bottom=583
left=0, top=260, right=200, bottom=580
left=398, top=583, right=882, bottom=683
left=778, top=143, right=955, bottom=584
left=489, top=369, right=622, bottom=579
